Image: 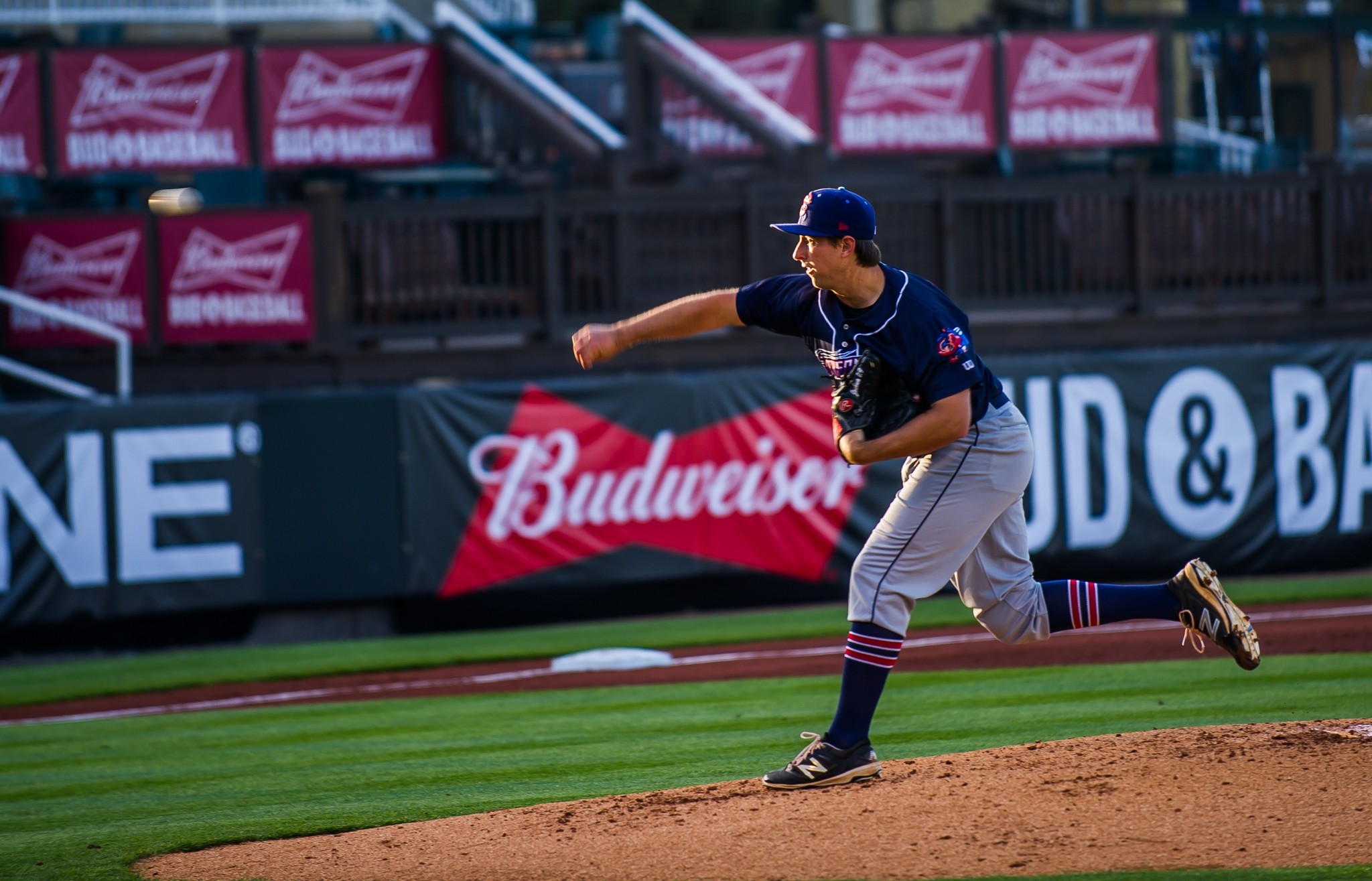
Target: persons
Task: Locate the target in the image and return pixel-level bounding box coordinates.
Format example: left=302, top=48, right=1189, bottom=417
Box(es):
left=571, top=186, right=1260, bottom=789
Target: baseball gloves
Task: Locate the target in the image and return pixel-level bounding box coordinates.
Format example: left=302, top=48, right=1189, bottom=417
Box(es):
left=819, top=350, right=879, bottom=467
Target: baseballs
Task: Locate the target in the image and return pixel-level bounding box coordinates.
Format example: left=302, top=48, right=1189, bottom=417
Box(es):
left=148, top=188, right=204, bottom=214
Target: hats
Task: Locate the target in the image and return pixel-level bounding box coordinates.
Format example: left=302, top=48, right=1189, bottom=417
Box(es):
left=770, top=186, right=877, bottom=242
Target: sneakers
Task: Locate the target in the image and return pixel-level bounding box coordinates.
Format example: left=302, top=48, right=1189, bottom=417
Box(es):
left=761, top=729, right=881, bottom=790
left=1165, top=557, right=1260, bottom=671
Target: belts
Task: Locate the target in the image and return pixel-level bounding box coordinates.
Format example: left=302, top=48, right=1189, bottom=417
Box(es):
left=991, top=393, right=1011, bottom=409
